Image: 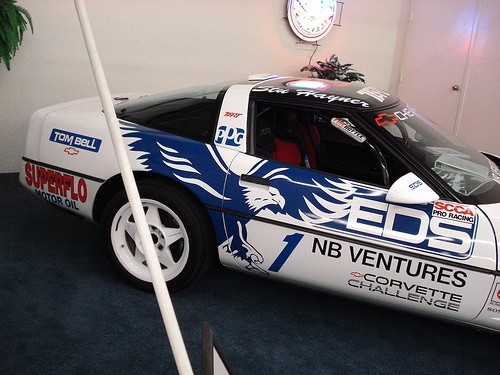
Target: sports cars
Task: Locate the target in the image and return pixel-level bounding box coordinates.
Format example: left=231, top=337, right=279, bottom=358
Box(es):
left=18, top=74, right=500, bottom=335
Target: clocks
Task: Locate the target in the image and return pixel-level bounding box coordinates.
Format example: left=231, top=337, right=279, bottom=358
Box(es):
left=287, top=0, right=336, bottom=42
